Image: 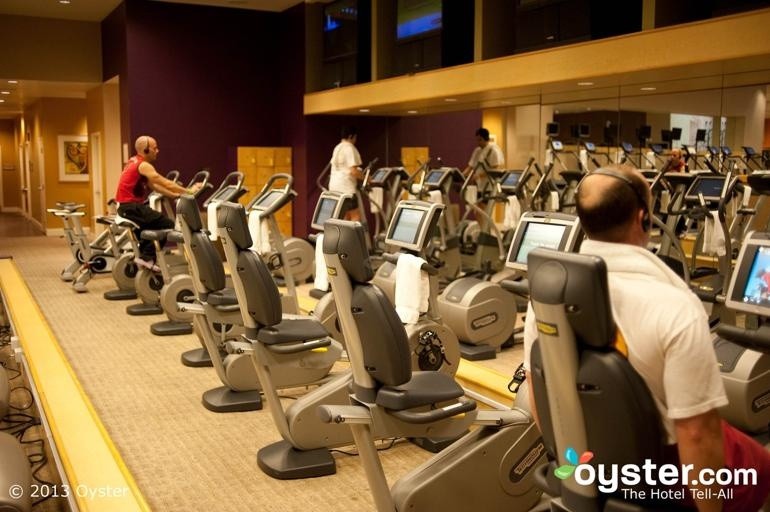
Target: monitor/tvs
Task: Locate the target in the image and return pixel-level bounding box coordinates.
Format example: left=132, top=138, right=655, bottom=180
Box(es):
left=370, top=169, right=770, bottom=201
left=515, top=221, right=567, bottom=264
left=256, top=191, right=285, bottom=207
left=391, top=208, right=425, bottom=244
left=730, top=244, right=770, bottom=308
left=545, top=122, right=757, bottom=155
left=215, top=188, right=236, bottom=199
left=313, top=198, right=338, bottom=226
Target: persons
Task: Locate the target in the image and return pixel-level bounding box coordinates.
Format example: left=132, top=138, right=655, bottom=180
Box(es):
left=115, top=134, right=205, bottom=259
left=666, top=148, right=690, bottom=174
left=329, top=124, right=371, bottom=223
left=519, top=162, right=770, bottom=512
left=459, top=127, right=505, bottom=201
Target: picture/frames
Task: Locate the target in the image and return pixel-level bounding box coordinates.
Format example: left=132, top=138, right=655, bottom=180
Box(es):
left=58, top=134, right=89, bottom=183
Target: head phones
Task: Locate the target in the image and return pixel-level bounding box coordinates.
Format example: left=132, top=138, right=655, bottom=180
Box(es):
left=144, top=137, right=149, bottom=153
left=590, top=169, right=650, bottom=232
